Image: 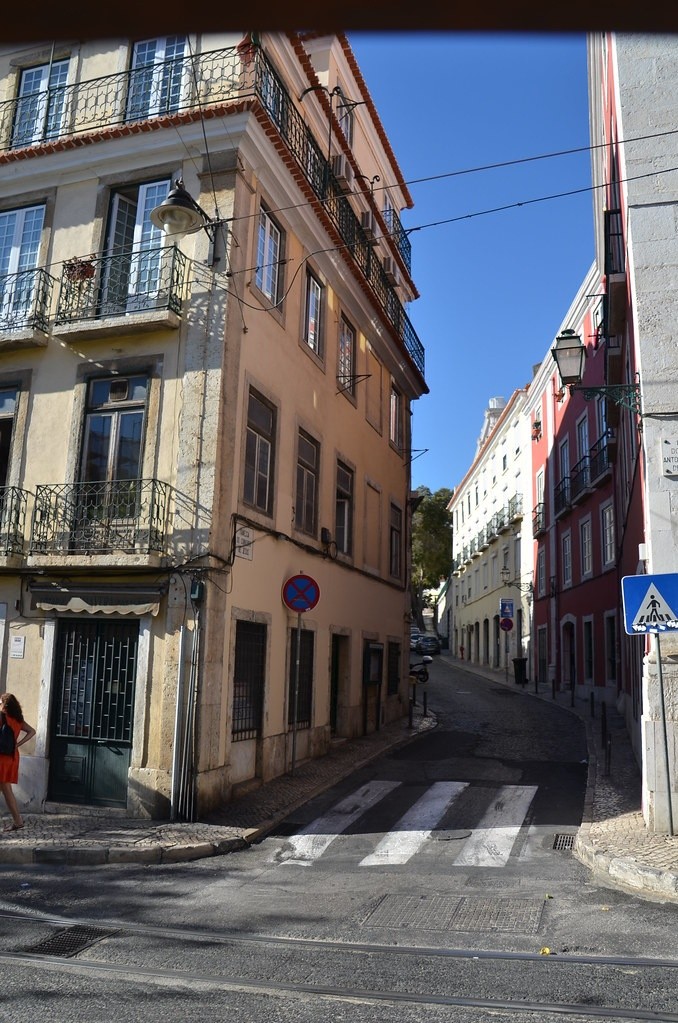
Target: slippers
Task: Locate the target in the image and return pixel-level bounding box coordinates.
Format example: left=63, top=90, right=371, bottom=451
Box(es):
left=4, top=823, right=24, bottom=831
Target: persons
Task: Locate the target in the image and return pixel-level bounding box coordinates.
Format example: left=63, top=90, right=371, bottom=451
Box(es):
left=0, top=693, right=37, bottom=832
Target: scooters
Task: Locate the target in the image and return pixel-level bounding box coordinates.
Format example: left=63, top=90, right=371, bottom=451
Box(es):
left=410, top=655, right=433, bottom=683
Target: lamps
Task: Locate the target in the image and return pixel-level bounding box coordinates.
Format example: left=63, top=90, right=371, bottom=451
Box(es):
left=149, top=176, right=224, bottom=266
left=550, top=328, right=643, bottom=432
left=500, top=565, right=534, bottom=593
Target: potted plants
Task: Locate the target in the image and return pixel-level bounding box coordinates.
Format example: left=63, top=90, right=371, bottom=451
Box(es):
left=63, top=253, right=97, bottom=281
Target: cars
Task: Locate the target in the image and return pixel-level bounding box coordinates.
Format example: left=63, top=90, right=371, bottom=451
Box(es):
left=411, top=633, right=427, bottom=651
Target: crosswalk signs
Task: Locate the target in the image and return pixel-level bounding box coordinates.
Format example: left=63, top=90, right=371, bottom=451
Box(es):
left=621, top=574, right=678, bottom=636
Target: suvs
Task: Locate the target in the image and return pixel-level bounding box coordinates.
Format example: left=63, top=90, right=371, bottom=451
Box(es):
left=417, top=637, right=441, bottom=655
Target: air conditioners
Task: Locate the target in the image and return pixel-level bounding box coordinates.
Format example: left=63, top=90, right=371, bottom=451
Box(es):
left=330, top=153, right=356, bottom=195
left=383, top=256, right=401, bottom=287
left=360, top=211, right=381, bottom=246
